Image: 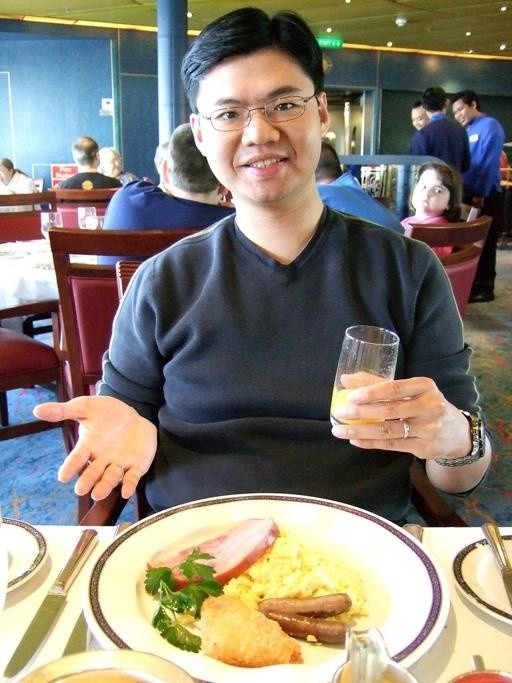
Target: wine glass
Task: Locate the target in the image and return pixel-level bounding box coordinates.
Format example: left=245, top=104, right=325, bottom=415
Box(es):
left=39, top=209, right=65, bottom=240
left=74, top=204, right=98, bottom=229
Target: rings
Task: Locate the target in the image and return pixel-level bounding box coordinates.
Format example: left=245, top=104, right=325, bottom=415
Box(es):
left=111, top=462, right=124, bottom=481
left=402, top=420, right=410, bottom=439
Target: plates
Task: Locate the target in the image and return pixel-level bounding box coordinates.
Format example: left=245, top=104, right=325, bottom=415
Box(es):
left=455, top=531, right=512, bottom=626
left=82, top=491, right=450, bottom=681
left=3, top=514, right=49, bottom=597
left=12, top=648, right=193, bottom=682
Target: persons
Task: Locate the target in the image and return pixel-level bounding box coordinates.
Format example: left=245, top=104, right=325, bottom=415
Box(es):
left=97, top=124, right=237, bottom=265
left=410, top=87, right=472, bottom=174
left=33, top=7, right=490, bottom=526
left=314, top=142, right=405, bottom=234
left=401, top=162, right=468, bottom=254
left=0, top=158, right=42, bottom=212
left=98, top=147, right=138, bottom=185
left=59, top=136, right=123, bottom=190
left=410, top=102, right=430, bottom=130
left=451, top=90, right=505, bottom=304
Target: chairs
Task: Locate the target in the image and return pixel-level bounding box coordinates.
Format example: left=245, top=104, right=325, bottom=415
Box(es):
left=405, top=216, right=493, bottom=321
left=74, top=259, right=472, bottom=526
left=0, top=322, right=76, bottom=479
left=0, top=192, right=57, bottom=245
left=49, top=224, right=220, bottom=525
left=52, top=188, right=117, bottom=233
left=34, top=179, right=44, bottom=188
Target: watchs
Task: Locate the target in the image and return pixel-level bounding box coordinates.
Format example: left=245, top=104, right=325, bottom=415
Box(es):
left=434, top=411, right=485, bottom=468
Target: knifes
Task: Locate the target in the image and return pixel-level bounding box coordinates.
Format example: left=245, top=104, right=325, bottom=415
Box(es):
left=481, top=521, right=512, bottom=606
left=4, top=520, right=102, bottom=677
left=61, top=520, right=136, bottom=656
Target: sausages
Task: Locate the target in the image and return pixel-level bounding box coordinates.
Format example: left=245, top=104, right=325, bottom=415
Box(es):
left=260, top=591, right=366, bottom=643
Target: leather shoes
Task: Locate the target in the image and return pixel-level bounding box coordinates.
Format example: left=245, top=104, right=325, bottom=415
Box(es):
left=471, top=291, right=494, bottom=302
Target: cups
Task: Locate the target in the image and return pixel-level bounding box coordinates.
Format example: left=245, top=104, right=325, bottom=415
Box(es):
left=329, top=324, right=401, bottom=447
left=452, top=666, right=512, bottom=682
left=85, top=214, right=106, bottom=228
left=330, top=625, right=418, bottom=682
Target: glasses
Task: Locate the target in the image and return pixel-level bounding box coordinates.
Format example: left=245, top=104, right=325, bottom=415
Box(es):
left=199, top=94, right=315, bottom=132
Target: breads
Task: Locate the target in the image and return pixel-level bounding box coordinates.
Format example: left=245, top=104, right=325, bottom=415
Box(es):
left=146, top=517, right=280, bottom=591
left=197, top=596, right=305, bottom=666
